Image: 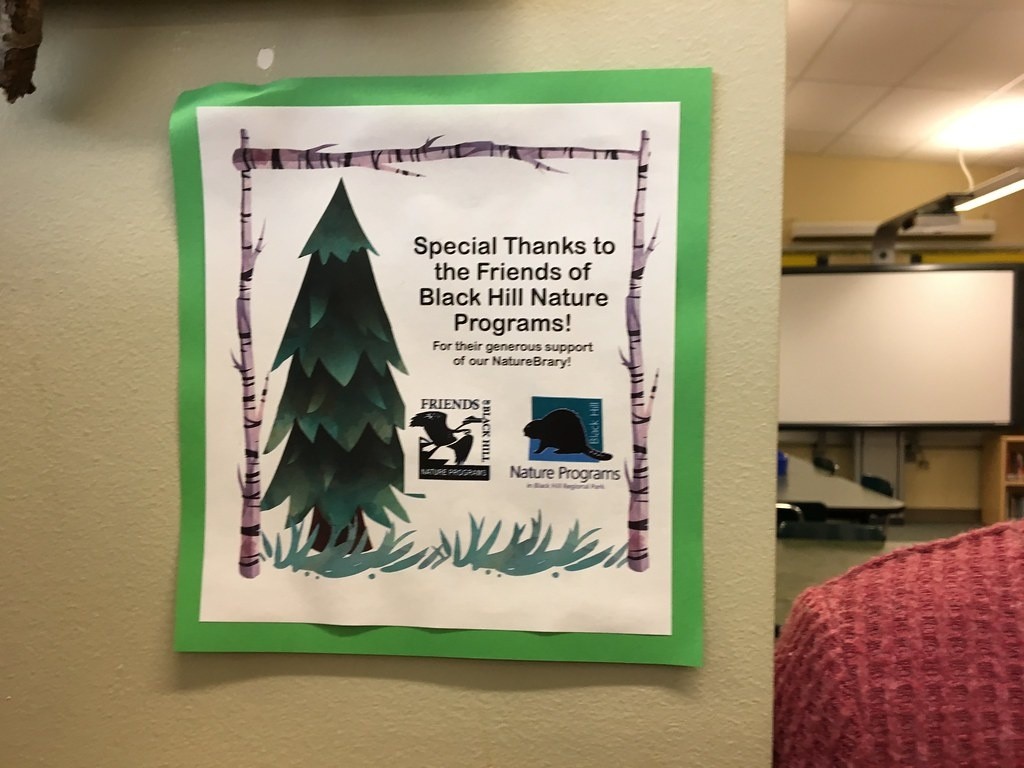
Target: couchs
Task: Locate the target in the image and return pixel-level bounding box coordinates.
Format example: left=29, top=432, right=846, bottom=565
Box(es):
left=774, top=515, right=1024, bottom=767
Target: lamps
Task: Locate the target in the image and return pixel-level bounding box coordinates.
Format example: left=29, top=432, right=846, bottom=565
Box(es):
left=951, top=169, right=1024, bottom=220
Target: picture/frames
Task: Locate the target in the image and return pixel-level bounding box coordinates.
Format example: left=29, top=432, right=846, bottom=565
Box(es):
left=173, top=68, right=713, bottom=668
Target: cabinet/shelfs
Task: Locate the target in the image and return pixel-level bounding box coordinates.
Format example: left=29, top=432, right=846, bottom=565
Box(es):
left=976, top=433, right=1024, bottom=524
